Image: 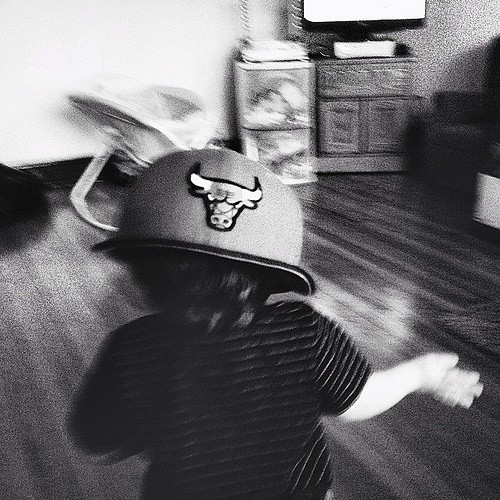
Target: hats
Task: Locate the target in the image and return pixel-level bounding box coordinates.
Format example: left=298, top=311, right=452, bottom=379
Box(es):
left=90, top=147, right=317, bottom=293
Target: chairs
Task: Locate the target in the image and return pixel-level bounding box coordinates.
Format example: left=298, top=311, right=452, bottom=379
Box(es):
left=63, top=86, right=224, bottom=240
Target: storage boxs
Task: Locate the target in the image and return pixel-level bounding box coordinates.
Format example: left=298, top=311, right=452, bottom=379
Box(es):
left=239, top=126, right=318, bottom=189
left=232, top=57, right=317, bottom=130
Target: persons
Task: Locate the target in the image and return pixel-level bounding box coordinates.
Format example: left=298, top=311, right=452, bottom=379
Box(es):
left=67, top=149, right=485, bottom=499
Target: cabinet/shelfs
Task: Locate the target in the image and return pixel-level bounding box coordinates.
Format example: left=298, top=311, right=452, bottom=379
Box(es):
left=315, top=60, right=420, bottom=174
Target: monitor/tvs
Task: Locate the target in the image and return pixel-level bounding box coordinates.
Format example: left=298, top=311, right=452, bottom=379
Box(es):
left=301, top=1, right=426, bottom=34
